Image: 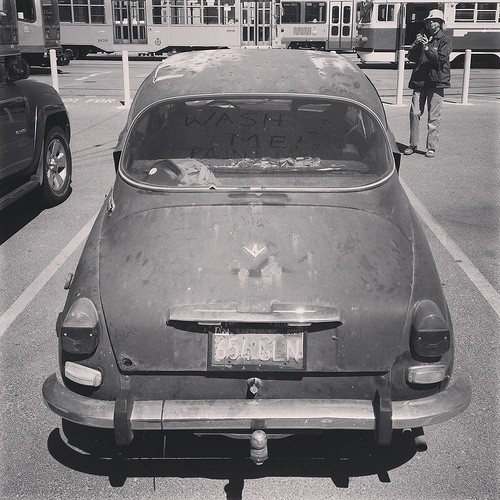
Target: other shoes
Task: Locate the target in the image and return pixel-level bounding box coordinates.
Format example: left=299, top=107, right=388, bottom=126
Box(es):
left=404, top=147, right=419, bottom=155
left=426, top=149, right=435, bottom=157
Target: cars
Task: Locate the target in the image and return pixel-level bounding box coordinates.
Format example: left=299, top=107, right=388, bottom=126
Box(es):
left=39, top=47, right=473, bottom=466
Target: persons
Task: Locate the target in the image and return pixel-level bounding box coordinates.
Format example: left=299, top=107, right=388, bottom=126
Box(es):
left=404, top=9, right=451, bottom=158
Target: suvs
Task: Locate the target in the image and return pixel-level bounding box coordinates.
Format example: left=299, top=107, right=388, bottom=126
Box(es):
left=0, top=54, right=73, bottom=215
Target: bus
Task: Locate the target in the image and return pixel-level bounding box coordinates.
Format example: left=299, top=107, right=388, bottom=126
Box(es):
left=354, top=0, right=500, bottom=67
left=43, top=0, right=472, bottom=59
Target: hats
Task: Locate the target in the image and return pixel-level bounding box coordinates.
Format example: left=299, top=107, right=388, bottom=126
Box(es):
left=424, top=9, right=445, bottom=24
